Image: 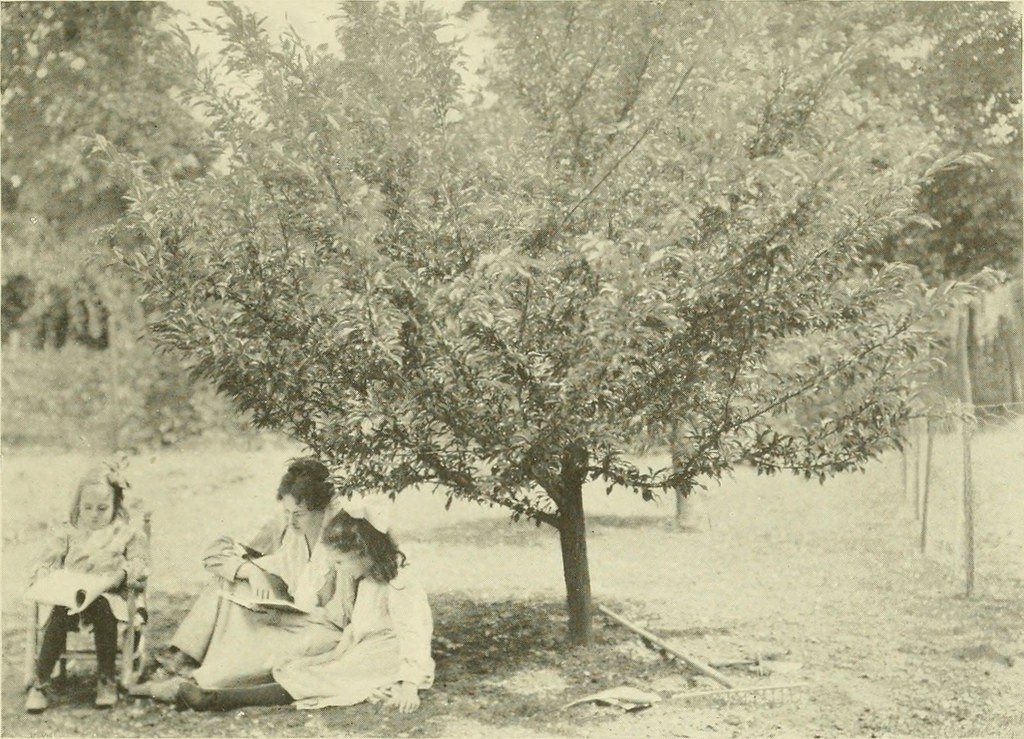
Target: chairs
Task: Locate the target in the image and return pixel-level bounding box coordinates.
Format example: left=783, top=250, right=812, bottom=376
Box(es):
left=21, top=497, right=153, bottom=699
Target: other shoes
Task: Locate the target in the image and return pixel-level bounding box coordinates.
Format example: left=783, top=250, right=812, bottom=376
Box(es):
left=129, top=674, right=173, bottom=697
left=25, top=683, right=50, bottom=711
left=178, top=683, right=228, bottom=712
left=151, top=676, right=199, bottom=703
left=95, top=676, right=118, bottom=706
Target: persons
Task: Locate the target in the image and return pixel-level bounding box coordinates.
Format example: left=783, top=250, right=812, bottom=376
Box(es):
left=126, top=460, right=358, bottom=702
left=178, top=504, right=436, bottom=715
left=19, top=461, right=149, bottom=713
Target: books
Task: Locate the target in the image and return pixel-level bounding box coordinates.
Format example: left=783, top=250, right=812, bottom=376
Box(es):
left=21, top=569, right=115, bottom=618
left=216, top=590, right=311, bottom=617
